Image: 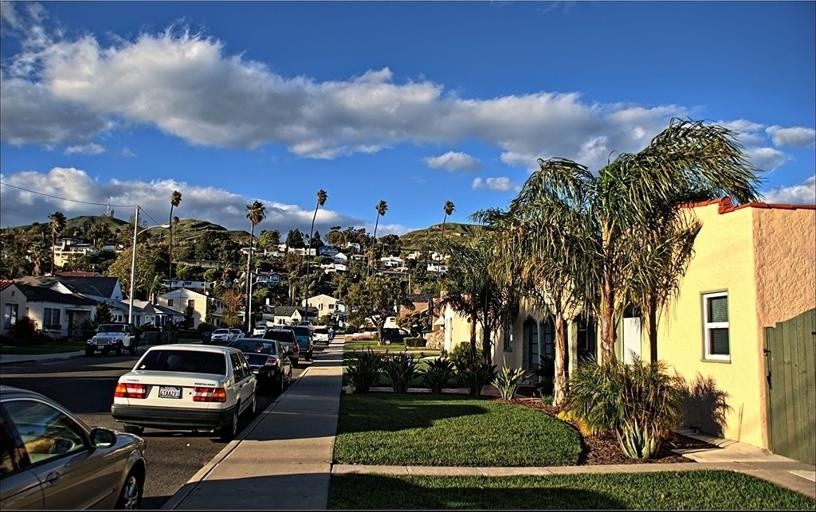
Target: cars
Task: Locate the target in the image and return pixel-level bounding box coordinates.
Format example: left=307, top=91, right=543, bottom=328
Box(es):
left=0, top=383, right=147, bottom=512
left=357, top=327, right=411, bottom=345
left=110, top=325, right=335, bottom=442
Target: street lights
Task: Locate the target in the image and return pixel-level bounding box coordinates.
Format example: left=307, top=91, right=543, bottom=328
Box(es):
left=247, top=279, right=267, bottom=333
left=127, top=223, right=172, bottom=323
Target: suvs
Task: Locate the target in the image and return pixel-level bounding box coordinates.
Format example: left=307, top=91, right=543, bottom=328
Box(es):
left=84, top=323, right=137, bottom=356
